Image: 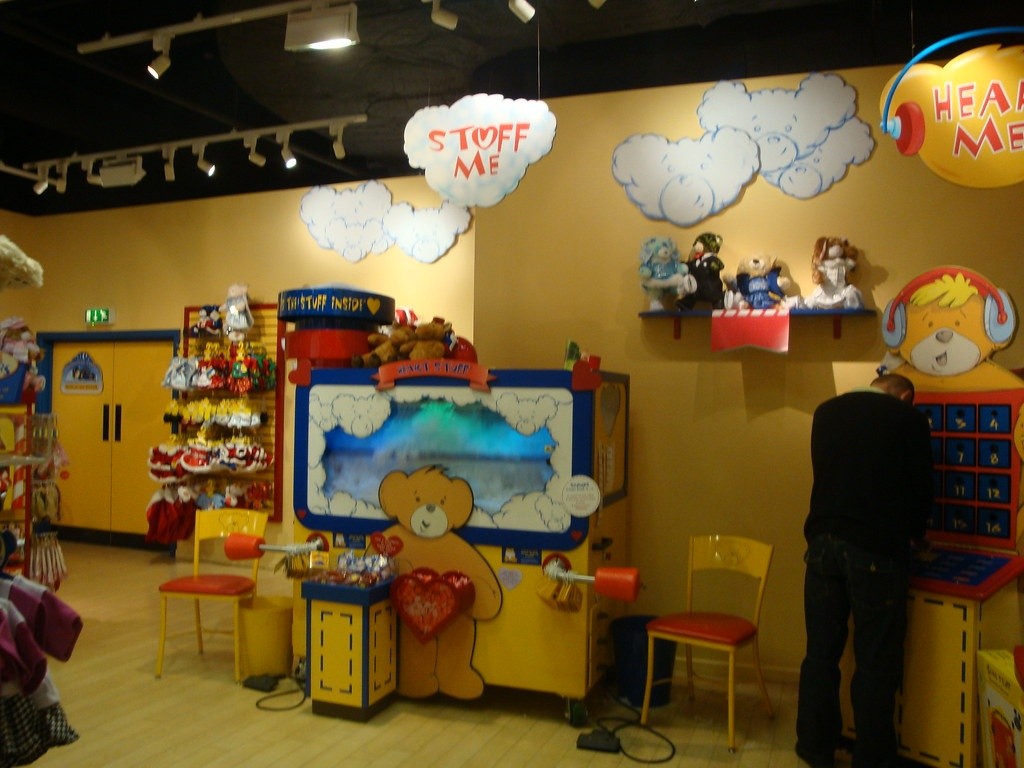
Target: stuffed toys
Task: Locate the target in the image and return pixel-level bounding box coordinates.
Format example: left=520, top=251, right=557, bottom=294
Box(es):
left=0, top=228, right=861, bottom=768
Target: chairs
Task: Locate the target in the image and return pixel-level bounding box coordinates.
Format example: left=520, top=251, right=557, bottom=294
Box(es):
left=155, top=507, right=269, bottom=681
left=637, top=534, right=773, bottom=754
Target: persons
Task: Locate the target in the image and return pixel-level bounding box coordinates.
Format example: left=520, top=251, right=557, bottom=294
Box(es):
left=795, top=373, right=934, bottom=768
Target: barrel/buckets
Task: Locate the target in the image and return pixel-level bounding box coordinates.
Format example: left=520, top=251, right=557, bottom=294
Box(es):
left=238, top=595, right=293, bottom=678
left=611, top=614, right=677, bottom=708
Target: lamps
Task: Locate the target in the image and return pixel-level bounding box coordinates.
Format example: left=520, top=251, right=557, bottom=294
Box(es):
left=276, top=131, right=297, bottom=169
left=508, top=0, right=535, bottom=23
left=244, top=135, right=266, bottom=167
left=192, top=144, right=216, bottom=176
left=162, top=148, right=175, bottom=182
left=99, top=153, right=146, bottom=188
left=431, top=0, right=459, bottom=31
left=330, top=122, right=346, bottom=159
left=147, top=34, right=176, bottom=78
left=284, top=0, right=361, bottom=53
left=33, top=166, right=49, bottom=195
left=81, top=160, right=102, bottom=186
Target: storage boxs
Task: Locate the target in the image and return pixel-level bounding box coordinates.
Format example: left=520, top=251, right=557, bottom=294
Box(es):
left=975, top=649, right=1024, bottom=768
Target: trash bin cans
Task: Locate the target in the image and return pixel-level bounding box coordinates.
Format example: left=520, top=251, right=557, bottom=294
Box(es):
left=235, top=596, right=293, bottom=676
left=607, top=615, right=676, bottom=708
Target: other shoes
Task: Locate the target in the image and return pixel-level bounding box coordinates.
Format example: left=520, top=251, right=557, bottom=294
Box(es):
left=853, top=753, right=911, bottom=768
left=795, top=740, right=834, bottom=768
